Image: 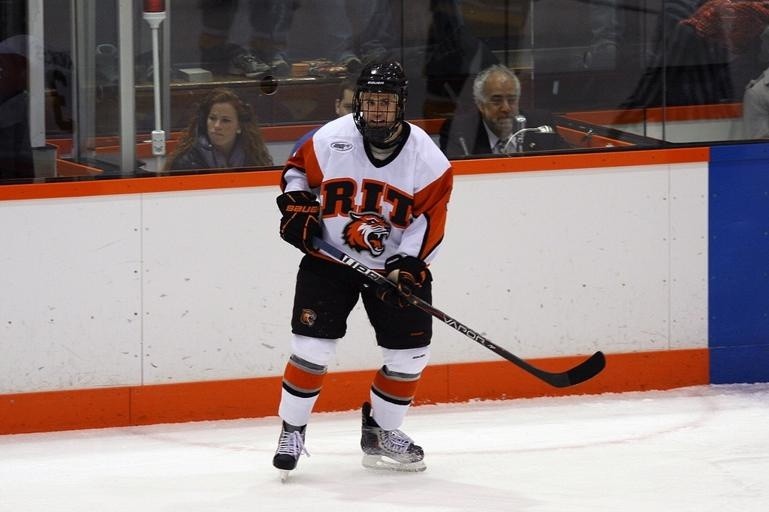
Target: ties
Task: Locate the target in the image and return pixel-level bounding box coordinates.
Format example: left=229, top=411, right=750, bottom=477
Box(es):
left=497, top=138, right=514, bottom=157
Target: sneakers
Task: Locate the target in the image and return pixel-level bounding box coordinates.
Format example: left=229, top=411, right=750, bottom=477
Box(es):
left=227, top=43, right=292, bottom=77
left=336, top=47, right=387, bottom=68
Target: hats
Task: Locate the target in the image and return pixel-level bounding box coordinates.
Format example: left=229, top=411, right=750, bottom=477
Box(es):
left=356, top=56, right=409, bottom=88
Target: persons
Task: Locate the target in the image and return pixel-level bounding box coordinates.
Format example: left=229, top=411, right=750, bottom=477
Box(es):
left=196, top=0, right=300, bottom=76
left=439, top=64, right=573, bottom=159
left=271, top=56, right=454, bottom=470
left=288, top=73, right=358, bottom=156
left=161, top=85, right=274, bottom=175
left=742, top=26, right=769, bottom=140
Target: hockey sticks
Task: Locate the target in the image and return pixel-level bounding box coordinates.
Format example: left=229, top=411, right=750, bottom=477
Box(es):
left=312, top=239, right=606, bottom=387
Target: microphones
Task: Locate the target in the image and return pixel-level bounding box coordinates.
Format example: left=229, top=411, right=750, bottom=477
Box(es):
left=512, top=114, right=526, bottom=151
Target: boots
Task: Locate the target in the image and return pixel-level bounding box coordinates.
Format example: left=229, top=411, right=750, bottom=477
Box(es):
left=273, top=419, right=307, bottom=470
left=360, top=401, right=424, bottom=463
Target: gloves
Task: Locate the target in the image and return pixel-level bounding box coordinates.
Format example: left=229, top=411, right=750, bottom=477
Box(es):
left=377, top=254, right=433, bottom=310
left=276, top=191, right=324, bottom=254
